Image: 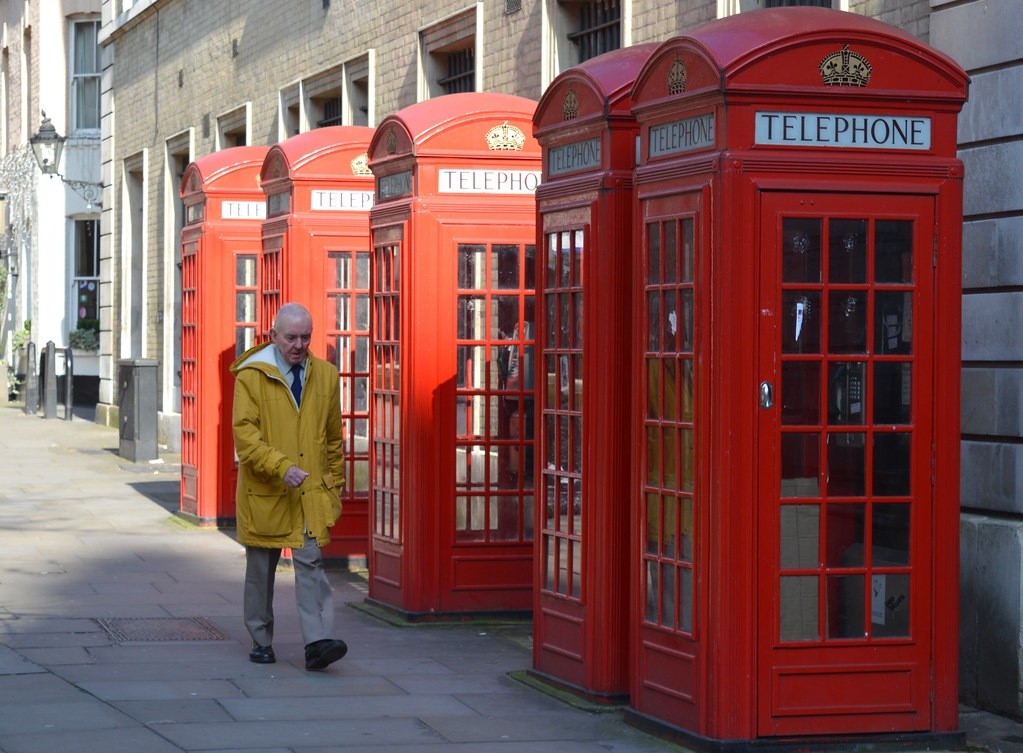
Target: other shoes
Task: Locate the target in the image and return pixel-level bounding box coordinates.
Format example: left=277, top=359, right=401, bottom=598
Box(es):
left=304, top=639, right=348, bottom=671
left=249, top=639, right=276, bottom=663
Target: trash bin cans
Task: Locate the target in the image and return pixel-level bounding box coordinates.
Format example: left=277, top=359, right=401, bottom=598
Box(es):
left=117, top=358, right=160, bottom=462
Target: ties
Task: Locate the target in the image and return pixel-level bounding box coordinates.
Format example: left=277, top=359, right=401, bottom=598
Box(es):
left=290, top=364, right=303, bottom=410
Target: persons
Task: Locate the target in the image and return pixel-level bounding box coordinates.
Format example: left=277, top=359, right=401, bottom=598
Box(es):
left=646, top=317, right=692, bottom=632
left=230, top=302, right=348, bottom=671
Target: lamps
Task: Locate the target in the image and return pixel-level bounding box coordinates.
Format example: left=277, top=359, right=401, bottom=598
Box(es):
left=27, top=108, right=67, bottom=176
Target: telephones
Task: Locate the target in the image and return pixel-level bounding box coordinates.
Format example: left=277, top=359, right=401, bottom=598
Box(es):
left=826, top=346, right=901, bottom=450
left=498, top=320, right=535, bottom=401
